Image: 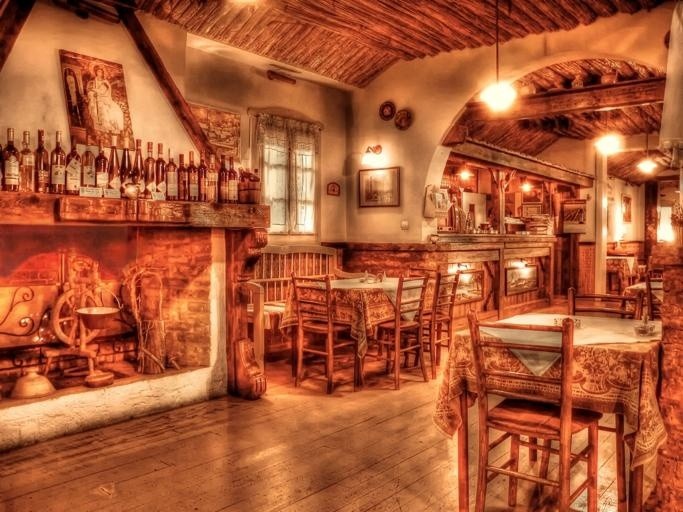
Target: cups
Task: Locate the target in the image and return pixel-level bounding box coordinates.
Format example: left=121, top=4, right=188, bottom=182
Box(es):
left=481, top=223, right=488, bottom=230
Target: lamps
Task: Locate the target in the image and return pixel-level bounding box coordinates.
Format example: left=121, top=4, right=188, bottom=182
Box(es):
left=517, top=176, right=533, bottom=195
left=474, top=0, right=519, bottom=114
left=636, top=119, right=659, bottom=176
left=361, top=144, right=381, bottom=165
left=456, top=164, right=474, bottom=182
left=591, top=109, right=627, bottom=158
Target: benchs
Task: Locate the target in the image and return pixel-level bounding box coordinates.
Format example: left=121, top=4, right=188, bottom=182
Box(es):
left=243, top=244, right=376, bottom=361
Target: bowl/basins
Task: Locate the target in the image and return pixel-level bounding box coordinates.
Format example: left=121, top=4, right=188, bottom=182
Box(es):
left=77, top=306, right=121, bottom=329
left=556, top=317, right=583, bottom=329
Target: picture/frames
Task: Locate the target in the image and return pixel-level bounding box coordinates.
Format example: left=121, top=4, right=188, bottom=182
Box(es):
left=621, top=193, right=632, bottom=223
left=521, top=202, right=543, bottom=219
left=357, top=167, right=401, bottom=207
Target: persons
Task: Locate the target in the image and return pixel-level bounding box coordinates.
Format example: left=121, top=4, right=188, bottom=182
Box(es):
left=63, top=68, right=86, bottom=127
left=86, top=62, right=123, bottom=135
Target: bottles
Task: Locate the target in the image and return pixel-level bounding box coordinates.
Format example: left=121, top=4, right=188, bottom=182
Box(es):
left=240, top=166, right=260, bottom=181
left=632, top=305, right=654, bottom=335
left=1, top=128, right=240, bottom=204
